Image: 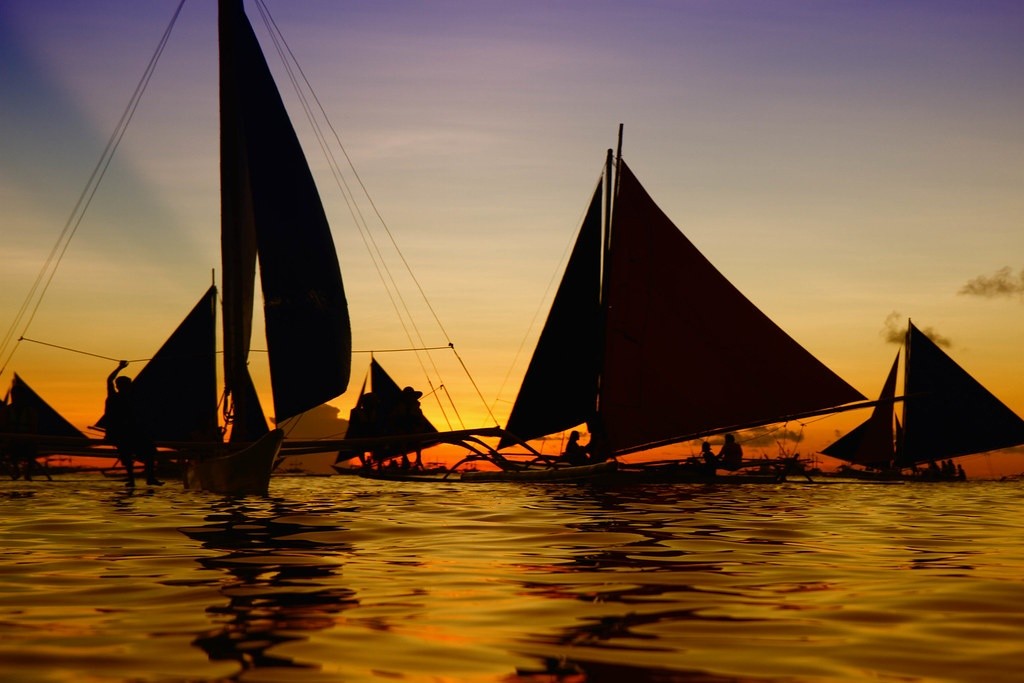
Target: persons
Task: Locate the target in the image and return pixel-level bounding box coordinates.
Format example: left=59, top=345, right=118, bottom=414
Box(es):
left=927, top=459, right=966, bottom=480
left=105, top=359, right=167, bottom=488
left=702, top=442, right=717, bottom=469
left=565, top=431, right=608, bottom=466
left=716, top=434, right=743, bottom=469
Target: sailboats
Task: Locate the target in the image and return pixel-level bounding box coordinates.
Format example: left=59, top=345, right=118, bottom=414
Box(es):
left=1, top=0, right=1023, bottom=496
